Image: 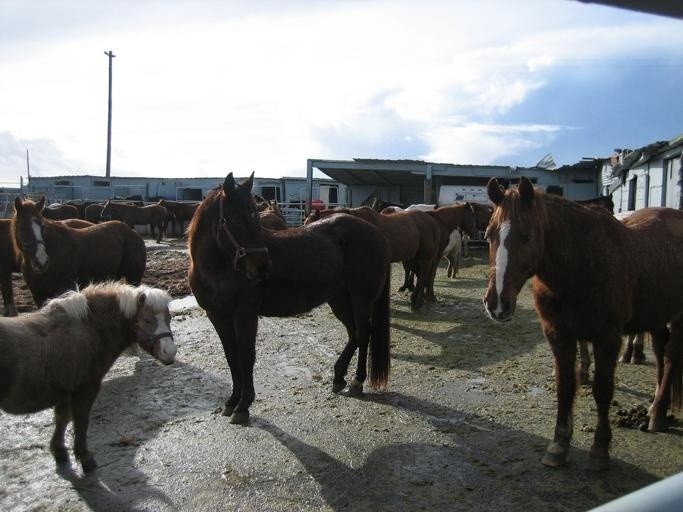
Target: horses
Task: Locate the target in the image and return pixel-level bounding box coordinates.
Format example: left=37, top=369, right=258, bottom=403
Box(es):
left=254, top=187, right=493, bottom=314
left=482, top=175, right=681, bottom=473
left=0, top=276, right=178, bottom=473
left=0, top=197, right=202, bottom=317
left=184, top=170, right=392, bottom=425
